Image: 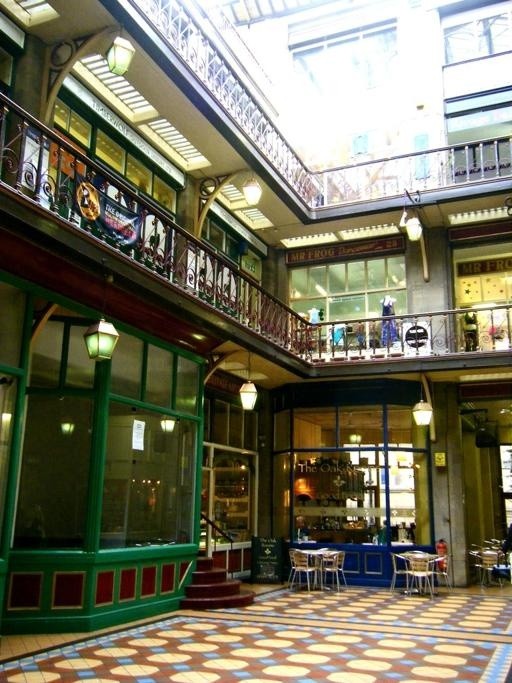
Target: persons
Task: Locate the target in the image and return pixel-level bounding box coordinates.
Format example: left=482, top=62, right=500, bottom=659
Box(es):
left=463, top=312, right=479, bottom=351
left=380, top=295, right=398, bottom=347
left=334, top=319, right=346, bottom=346
left=307, top=307, right=320, bottom=324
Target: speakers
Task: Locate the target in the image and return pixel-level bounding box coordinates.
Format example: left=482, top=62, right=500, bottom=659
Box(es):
left=475, top=420, right=498, bottom=448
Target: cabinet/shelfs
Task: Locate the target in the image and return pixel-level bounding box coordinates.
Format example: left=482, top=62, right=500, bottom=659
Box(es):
left=201, top=466, right=250, bottom=534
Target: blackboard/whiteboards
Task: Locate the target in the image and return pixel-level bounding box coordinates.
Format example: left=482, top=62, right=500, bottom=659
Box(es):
left=251, top=537, right=284, bottom=582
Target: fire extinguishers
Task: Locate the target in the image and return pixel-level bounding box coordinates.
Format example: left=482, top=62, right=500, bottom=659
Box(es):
left=437, top=538, right=447, bottom=570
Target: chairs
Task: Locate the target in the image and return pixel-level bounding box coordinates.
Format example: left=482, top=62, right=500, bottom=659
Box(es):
left=285, top=546, right=347, bottom=593
left=467, top=538, right=508, bottom=588
left=388, top=550, right=453, bottom=600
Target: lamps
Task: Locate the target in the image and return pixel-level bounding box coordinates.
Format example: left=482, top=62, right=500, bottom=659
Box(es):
left=405, top=208, right=424, bottom=242
left=237, top=350, right=258, bottom=413
left=241, top=170, right=263, bottom=206
left=103, top=22, right=136, bottom=77
left=86, top=310, right=120, bottom=361
left=411, top=371, right=434, bottom=427
